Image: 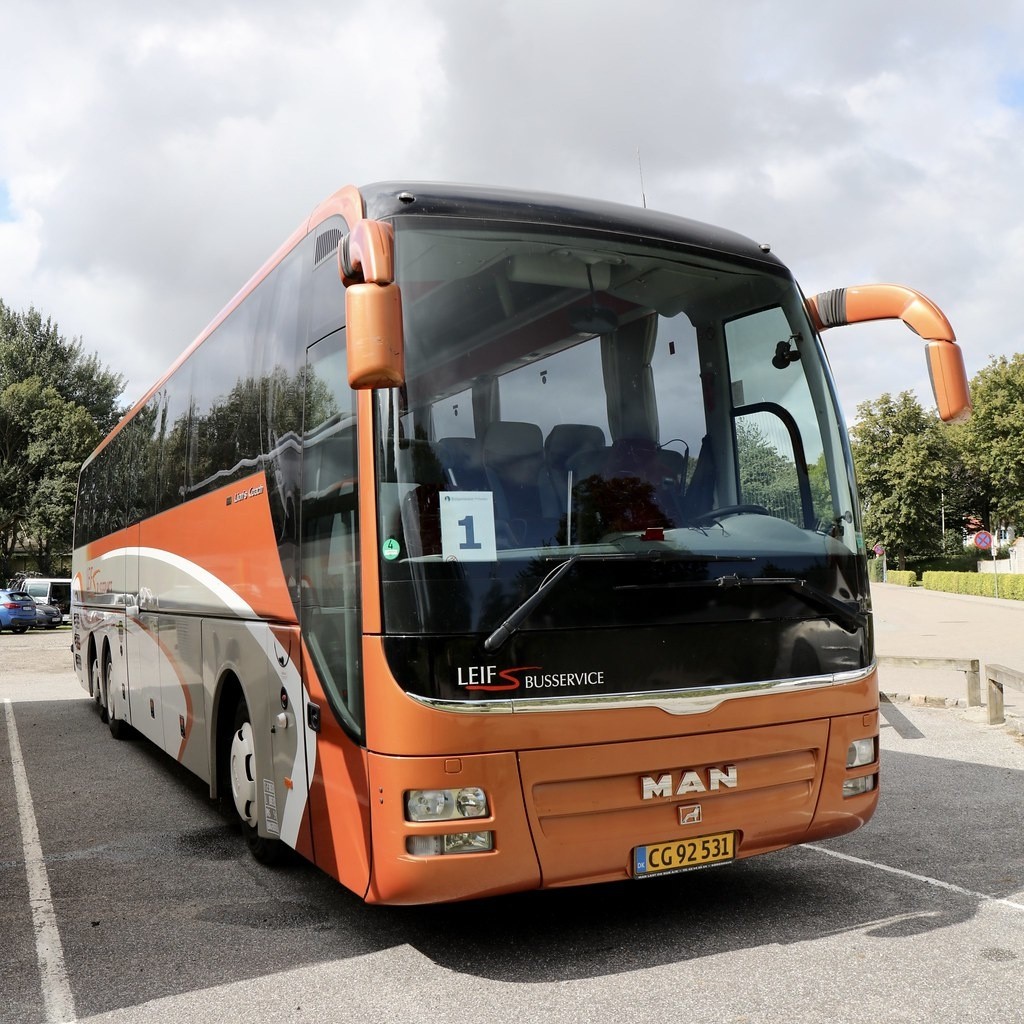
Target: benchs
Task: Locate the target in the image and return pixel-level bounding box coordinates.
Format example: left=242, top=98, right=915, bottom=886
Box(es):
left=876, top=654, right=982, bottom=707
left=985, top=663, right=1024, bottom=726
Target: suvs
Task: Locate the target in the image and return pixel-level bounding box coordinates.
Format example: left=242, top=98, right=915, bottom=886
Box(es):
left=0, top=588, right=39, bottom=634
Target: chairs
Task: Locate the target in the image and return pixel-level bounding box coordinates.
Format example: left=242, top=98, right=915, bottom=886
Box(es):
left=475, top=421, right=545, bottom=530
left=436, top=436, right=478, bottom=470
left=545, top=424, right=605, bottom=523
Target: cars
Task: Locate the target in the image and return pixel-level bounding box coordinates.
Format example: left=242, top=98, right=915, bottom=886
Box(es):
left=28, top=594, right=63, bottom=627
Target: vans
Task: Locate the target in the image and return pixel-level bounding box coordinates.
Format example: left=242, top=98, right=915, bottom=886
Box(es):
left=21, top=578, right=72, bottom=622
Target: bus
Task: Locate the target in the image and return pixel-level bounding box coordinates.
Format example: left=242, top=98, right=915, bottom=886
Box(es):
left=70, top=174, right=976, bottom=910
